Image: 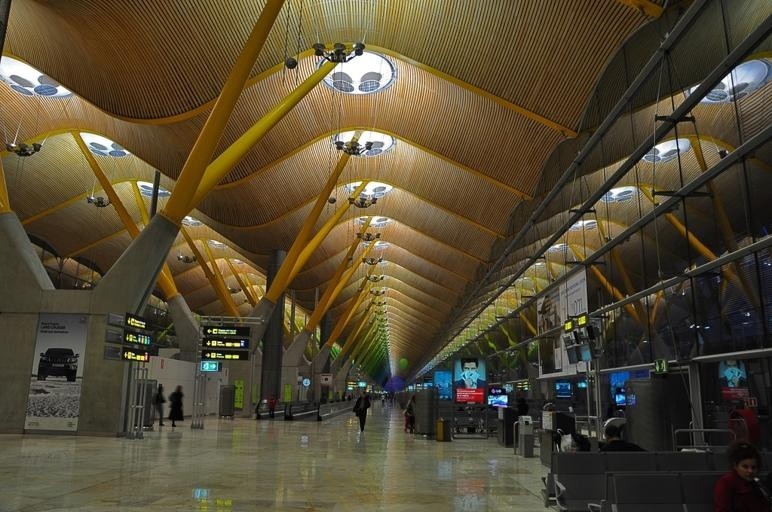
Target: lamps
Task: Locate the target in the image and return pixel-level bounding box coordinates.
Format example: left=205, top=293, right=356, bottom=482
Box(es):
left=207, top=239, right=230, bottom=251
left=318, top=49, right=398, bottom=157
left=177, top=214, right=204, bottom=265
left=1, top=53, right=74, bottom=159
left=330, top=124, right=398, bottom=210
left=344, top=176, right=396, bottom=242
left=79, top=131, right=132, bottom=209
left=136, top=180, right=173, bottom=200
left=308, top=0, right=375, bottom=63
left=353, top=212, right=392, bottom=356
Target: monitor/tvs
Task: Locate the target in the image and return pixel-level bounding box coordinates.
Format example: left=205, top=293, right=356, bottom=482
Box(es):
left=488, top=395, right=508, bottom=406
left=614, top=394, right=636, bottom=407
left=553, top=380, right=574, bottom=400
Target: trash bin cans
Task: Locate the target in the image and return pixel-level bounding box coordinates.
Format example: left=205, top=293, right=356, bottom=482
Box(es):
left=436, top=416, right=451, bottom=442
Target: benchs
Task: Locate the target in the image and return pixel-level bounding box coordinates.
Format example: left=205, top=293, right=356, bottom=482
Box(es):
left=542, top=449, right=772, bottom=512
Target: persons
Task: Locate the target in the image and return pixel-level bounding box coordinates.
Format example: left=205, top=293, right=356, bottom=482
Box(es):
left=454, top=358, right=486, bottom=388
left=268, top=395, right=277, bottom=420
left=713, top=436, right=772, bottom=512
left=321, top=388, right=416, bottom=434
left=717, top=359, right=747, bottom=388
left=516, top=397, right=530, bottom=416
left=151, top=386, right=166, bottom=426
left=598, top=423, right=647, bottom=452
left=166, top=384, right=185, bottom=427
